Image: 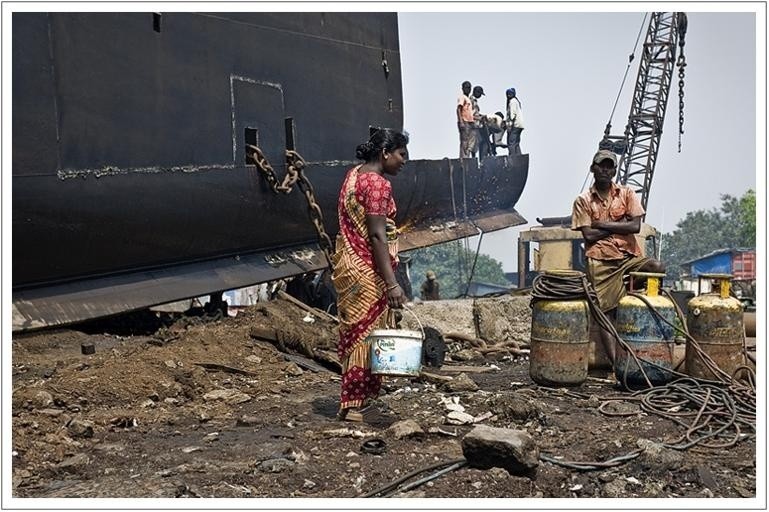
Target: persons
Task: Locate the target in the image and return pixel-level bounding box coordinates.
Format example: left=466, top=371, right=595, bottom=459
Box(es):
left=457, top=81, right=524, bottom=158
left=571, top=149, right=665, bottom=380
left=329, top=128, right=409, bottom=424
left=420, top=271, right=440, bottom=300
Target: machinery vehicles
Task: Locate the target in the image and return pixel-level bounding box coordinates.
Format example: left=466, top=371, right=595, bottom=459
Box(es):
left=509, top=11, right=690, bottom=292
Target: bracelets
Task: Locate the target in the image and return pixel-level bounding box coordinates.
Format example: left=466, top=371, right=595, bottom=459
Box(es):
left=386, top=284, right=399, bottom=292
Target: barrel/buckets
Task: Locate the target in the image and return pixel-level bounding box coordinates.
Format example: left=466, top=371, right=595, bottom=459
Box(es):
left=366, top=303, right=427, bottom=377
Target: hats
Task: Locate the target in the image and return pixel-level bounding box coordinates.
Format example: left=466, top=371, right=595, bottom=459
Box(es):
left=593, top=149, right=617, bottom=167
left=426, top=271, right=435, bottom=280
left=474, top=86, right=485, bottom=96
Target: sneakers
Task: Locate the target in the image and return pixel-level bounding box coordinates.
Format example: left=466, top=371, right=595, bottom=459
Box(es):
left=345, top=404, right=395, bottom=424
